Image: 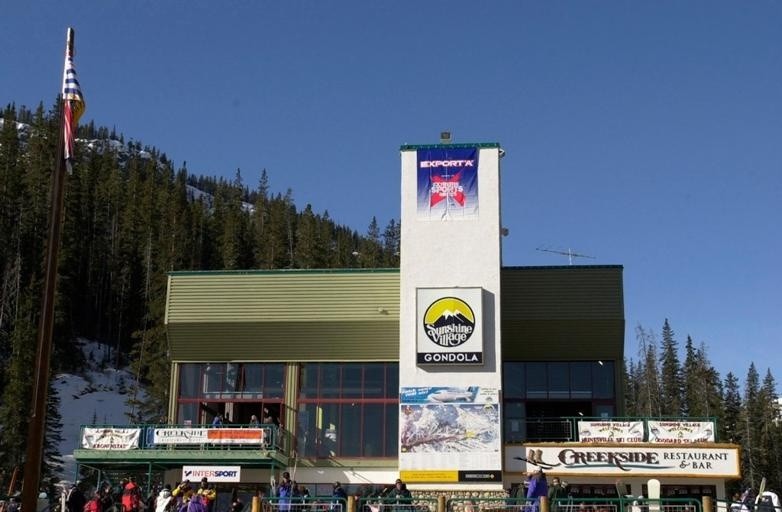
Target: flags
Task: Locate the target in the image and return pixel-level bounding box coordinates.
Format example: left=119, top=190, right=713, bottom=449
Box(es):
left=62, top=34, right=86, bottom=175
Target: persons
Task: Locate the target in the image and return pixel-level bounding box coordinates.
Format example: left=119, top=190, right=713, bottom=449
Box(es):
left=730, top=480, right=781, bottom=511
left=332, top=478, right=413, bottom=512
left=32, top=475, right=216, bottom=512
left=157, top=405, right=280, bottom=445
left=515, top=465, right=568, bottom=512
left=276, top=472, right=325, bottom=512
left=232, top=497, right=244, bottom=512
